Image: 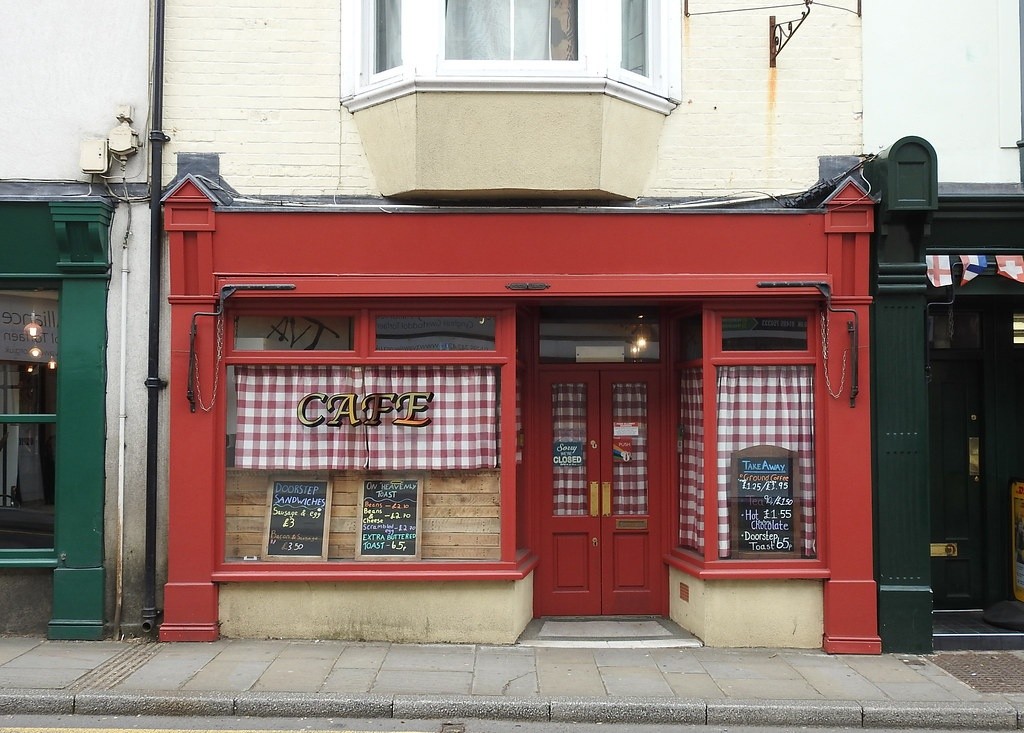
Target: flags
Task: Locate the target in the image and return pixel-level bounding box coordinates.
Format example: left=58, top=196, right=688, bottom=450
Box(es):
left=926, top=254, right=953, bottom=287
left=959, top=254, right=988, bottom=286
left=995, top=255, right=1024, bottom=282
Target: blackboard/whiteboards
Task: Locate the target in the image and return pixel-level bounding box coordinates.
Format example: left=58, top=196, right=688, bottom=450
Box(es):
left=728, top=444, right=805, bottom=561
left=260, top=474, right=334, bottom=563
left=353, top=471, right=424, bottom=563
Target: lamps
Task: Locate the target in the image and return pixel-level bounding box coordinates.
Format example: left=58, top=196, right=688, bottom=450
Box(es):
left=628, top=323, right=653, bottom=359
left=48, top=352, right=58, bottom=369
left=28, top=340, right=43, bottom=360
left=22, top=303, right=42, bottom=340
